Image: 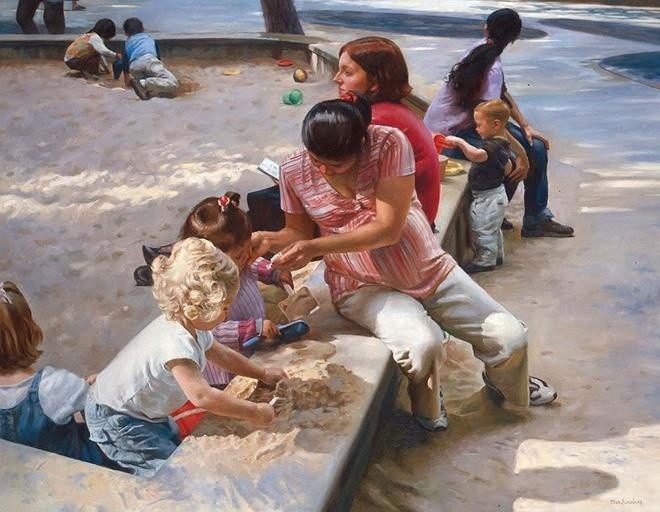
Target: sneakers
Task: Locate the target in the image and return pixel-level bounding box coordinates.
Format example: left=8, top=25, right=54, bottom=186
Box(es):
left=83, top=70, right=98, bottom=80
left=500, top=217, right=512, bottom=228
left=131, top=77, right=149, bottom=101
left=482, top=371, right=558, bottom=405
left=464, top=263, right=496, bottom=273
left=409, top=386, right=448, bottom=431
left=497, top=255, right=502, bottom=264
left=521, top=220, right=574, bottom=237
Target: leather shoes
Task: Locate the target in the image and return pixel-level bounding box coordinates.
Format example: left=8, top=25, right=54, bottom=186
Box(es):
left=134, top=266, right=154, bottom=286
left=142, top=244, right=159, bottom=263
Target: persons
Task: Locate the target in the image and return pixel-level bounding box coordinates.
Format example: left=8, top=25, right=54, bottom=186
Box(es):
left=183, top=192, right=293, bottom=393
left=85, top=237, right=288, bottom=476
left=64, top=20, right=121, bottom=78
left=15, top=0, right=67, bottom=34
left=125, top=18, right=179, bottom=99
left=444, top=99, right=511, bottom=271
left=424, top=8, right=576, bottom=239
left=134, top=36, right=441, bottom=286
left=0, top=281, right=109, bottom=467
left=250, top=90, right=556, bottom=432
